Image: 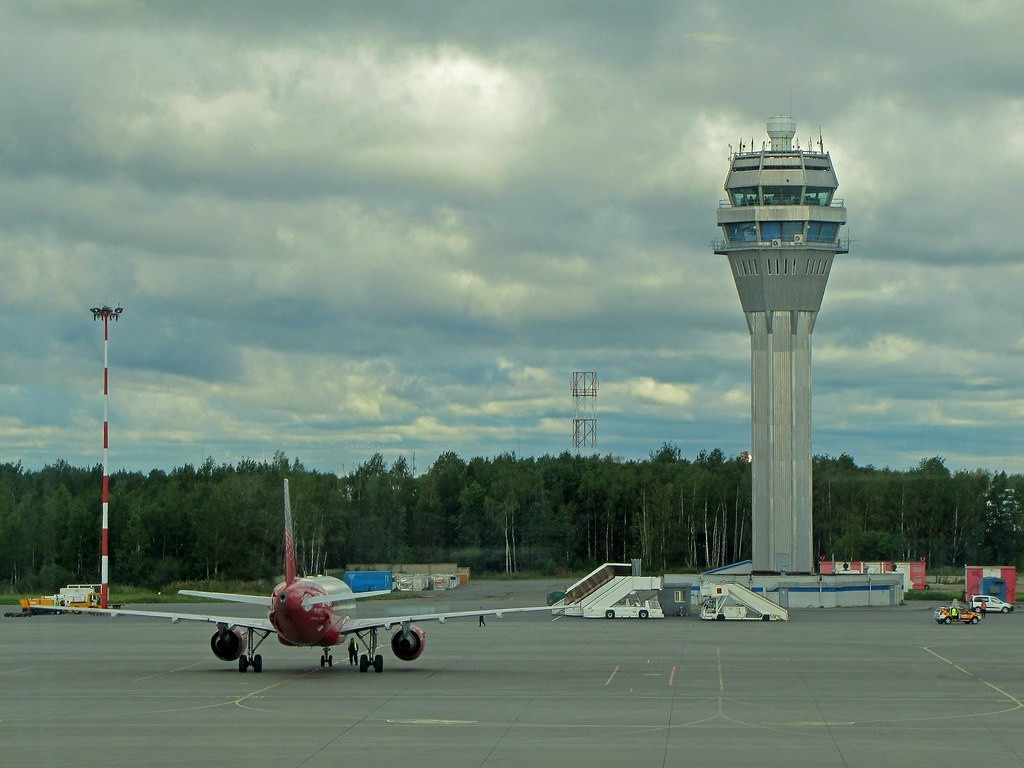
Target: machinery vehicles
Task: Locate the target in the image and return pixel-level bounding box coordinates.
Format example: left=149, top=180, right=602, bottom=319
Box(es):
left=699, top=580, right=790, bottom=622
left=583, top=576, right=665, bottom=619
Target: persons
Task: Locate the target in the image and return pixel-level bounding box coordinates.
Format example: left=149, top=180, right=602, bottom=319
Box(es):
left=348, top=638, right=360, bottom=666
left=981, top=600, right=986, bottom=618
left=478, top=607, right=486, bottom=627
left=55, top=598, right=65, bottom=606
left=952, top=606, right=957, bottom=624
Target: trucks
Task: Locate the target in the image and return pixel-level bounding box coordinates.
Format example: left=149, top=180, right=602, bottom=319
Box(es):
left=65, top=592, right=103, bottom=614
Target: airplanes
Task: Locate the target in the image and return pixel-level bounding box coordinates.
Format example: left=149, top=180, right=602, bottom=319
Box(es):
left=27, top=477, right=582, bottom=673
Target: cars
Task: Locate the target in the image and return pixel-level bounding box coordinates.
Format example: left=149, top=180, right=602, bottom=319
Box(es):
left=934, top=604, right=982, bottom=625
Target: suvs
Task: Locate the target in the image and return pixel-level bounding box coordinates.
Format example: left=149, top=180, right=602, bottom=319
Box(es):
left=969, top=594, right=1014, bottom=613
